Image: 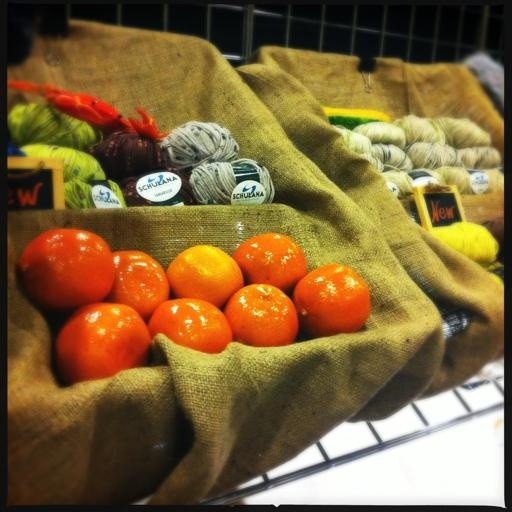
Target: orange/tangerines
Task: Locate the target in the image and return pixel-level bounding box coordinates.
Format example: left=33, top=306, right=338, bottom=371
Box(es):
left=21, top=228, right=371, bottom=381
